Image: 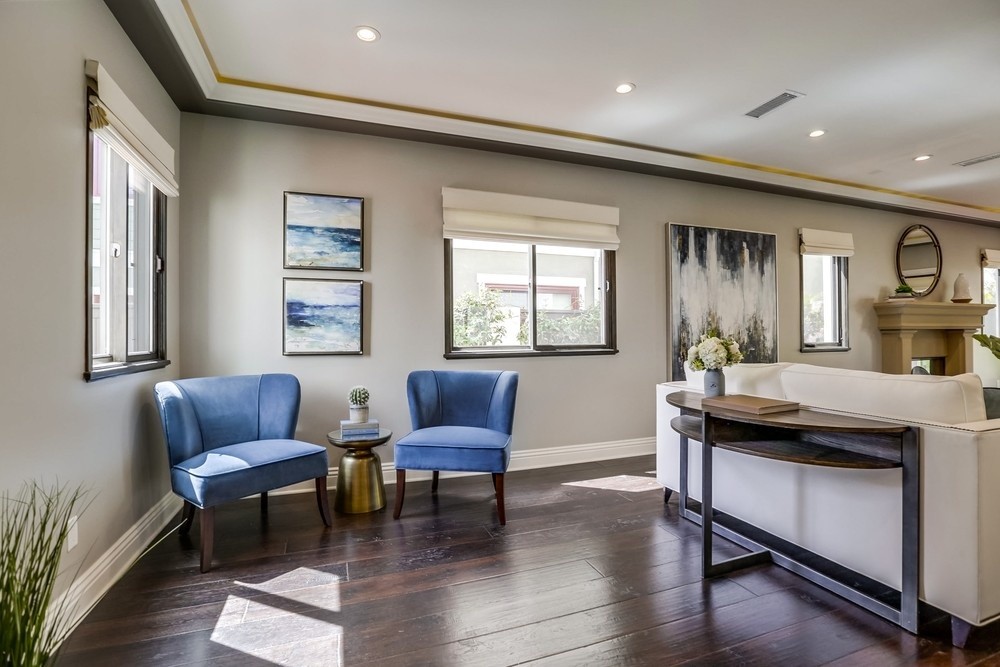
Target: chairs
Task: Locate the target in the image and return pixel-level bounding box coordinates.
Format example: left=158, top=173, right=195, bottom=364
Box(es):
left=152, top=373, right=333, bottom=573
left=392, top=369, right=519, bottom=525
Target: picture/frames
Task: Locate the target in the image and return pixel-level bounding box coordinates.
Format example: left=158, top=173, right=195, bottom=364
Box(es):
left=283, top=191, right=364, bottom=271
left=664, top=222, right=780, bottom=382
left=283, top=276, right=364, bottom=356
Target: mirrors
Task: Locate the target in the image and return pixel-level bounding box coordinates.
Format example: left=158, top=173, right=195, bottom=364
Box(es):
left=896, top=224, right=943, bottom=298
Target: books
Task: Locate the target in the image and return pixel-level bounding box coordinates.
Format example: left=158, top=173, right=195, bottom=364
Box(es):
left=702, top=394, right=799, bottom=415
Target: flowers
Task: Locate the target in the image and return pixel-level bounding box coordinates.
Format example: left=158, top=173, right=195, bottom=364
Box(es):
left=686, top=324, right=744, bottom=372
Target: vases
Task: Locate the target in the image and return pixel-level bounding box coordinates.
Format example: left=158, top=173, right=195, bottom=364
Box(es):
left=704, top=368, right=725, bottom=398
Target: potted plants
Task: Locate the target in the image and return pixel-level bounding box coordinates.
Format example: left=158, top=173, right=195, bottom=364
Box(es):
left=894, top=283, right=915, bottom=297
left=348, top=387, right=371, bottom=422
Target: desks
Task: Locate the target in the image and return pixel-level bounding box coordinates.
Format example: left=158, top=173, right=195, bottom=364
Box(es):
left=666, top=390, right=923, bottom=635
left=327, top=426, right=395, bottom=515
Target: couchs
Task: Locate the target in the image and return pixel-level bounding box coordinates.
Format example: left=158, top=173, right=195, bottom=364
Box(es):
left=655, top=357, right=1000, bottom=649
